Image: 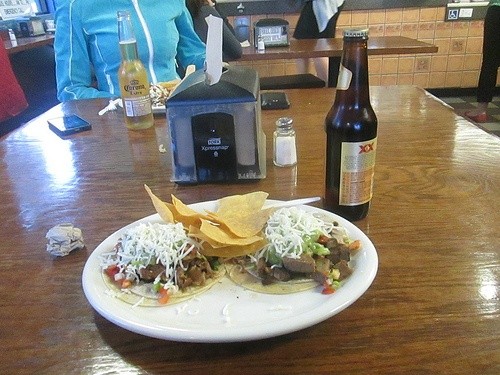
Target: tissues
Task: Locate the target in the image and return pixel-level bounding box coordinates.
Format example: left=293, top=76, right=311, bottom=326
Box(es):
left=157, top=14, right=267, bottom=186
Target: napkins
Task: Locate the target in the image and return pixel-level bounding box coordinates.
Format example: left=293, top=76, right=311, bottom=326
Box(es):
left=45, top=223, right=85, bottom=258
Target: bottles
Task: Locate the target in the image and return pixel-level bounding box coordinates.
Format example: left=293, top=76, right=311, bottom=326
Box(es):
left=7, top=29, right=16, bottom=41
left=257, top=35, right=265, bottom=54
left=274, top=117, right=298, bottom=167
left=117, top=10, right=155, bottom=130
left=234, top=3, right=251, bottom=48
left=324, top=27, right=377, bottom=220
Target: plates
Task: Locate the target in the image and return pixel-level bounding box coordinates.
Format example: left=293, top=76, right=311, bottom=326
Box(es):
left=118, top=97, right=167, bottom=115
left=81, top=198, right=379, bottom=342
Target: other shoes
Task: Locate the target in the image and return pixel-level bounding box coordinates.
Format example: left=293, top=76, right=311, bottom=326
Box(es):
left=464, top=111, right=487, bottom=123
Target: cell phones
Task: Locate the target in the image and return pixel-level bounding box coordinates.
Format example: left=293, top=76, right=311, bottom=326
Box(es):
left=261, top=91, right=290, bottom=110
left=46, top=113, right=92, bottom=137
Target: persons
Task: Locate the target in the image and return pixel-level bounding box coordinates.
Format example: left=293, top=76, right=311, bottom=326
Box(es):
left=53, top=0, right=230, bottom=103
left=175, top=0, right=242, bottom=80
left=464, top=0, right=500, bottom=123
left=293, top=0, right=345, bottom=87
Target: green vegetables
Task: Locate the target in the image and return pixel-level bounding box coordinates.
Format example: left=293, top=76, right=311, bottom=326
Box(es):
left=264, top=230, right=352, bottom=291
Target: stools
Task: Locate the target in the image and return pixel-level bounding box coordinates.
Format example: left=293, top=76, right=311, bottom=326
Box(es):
left=259, top=72, right=326, bottom=91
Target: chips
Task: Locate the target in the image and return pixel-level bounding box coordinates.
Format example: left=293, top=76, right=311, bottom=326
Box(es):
left=143, top=185, right=267, bottom=257
left=158, top=64, right=194, bottom=91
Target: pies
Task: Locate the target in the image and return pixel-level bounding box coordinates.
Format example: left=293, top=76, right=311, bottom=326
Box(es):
left=102, top=265, right=226, bottom=306
left=225, top=259, right=321, bottom=294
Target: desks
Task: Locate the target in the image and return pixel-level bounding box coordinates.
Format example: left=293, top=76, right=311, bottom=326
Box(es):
left=0, top=84, right=500, bottom=375
left=5, top=34, right=55, bottom=54
left=223, top=35, right=439, bottom=89
